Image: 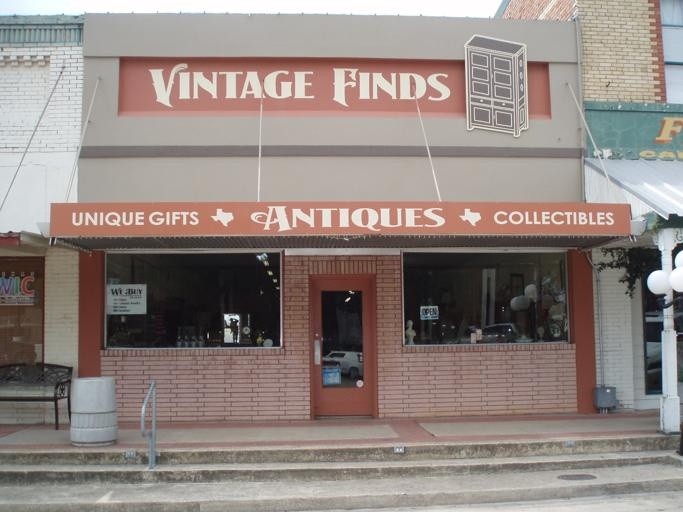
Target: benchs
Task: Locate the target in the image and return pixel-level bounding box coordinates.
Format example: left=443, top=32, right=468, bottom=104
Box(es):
left=0, top=362, right=73, bottom=430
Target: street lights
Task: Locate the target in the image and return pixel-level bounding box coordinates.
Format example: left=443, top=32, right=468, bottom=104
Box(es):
left=509, top=285, right=552, bottom=343
left=646, top=251, right=682, bottom=456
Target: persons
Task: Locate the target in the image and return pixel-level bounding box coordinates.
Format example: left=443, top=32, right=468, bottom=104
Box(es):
left=230, top=318, right=238, bottom=343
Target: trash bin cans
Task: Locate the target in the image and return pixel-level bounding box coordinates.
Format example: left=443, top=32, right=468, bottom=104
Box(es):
left=70, top=376, right=118, bottom=448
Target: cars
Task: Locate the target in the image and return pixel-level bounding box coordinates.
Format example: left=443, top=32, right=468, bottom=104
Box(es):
left=322, top=350, right=362, bottom=380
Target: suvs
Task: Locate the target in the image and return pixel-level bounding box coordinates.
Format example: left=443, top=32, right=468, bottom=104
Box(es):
left=459, top=323, right=521, bottom=344
